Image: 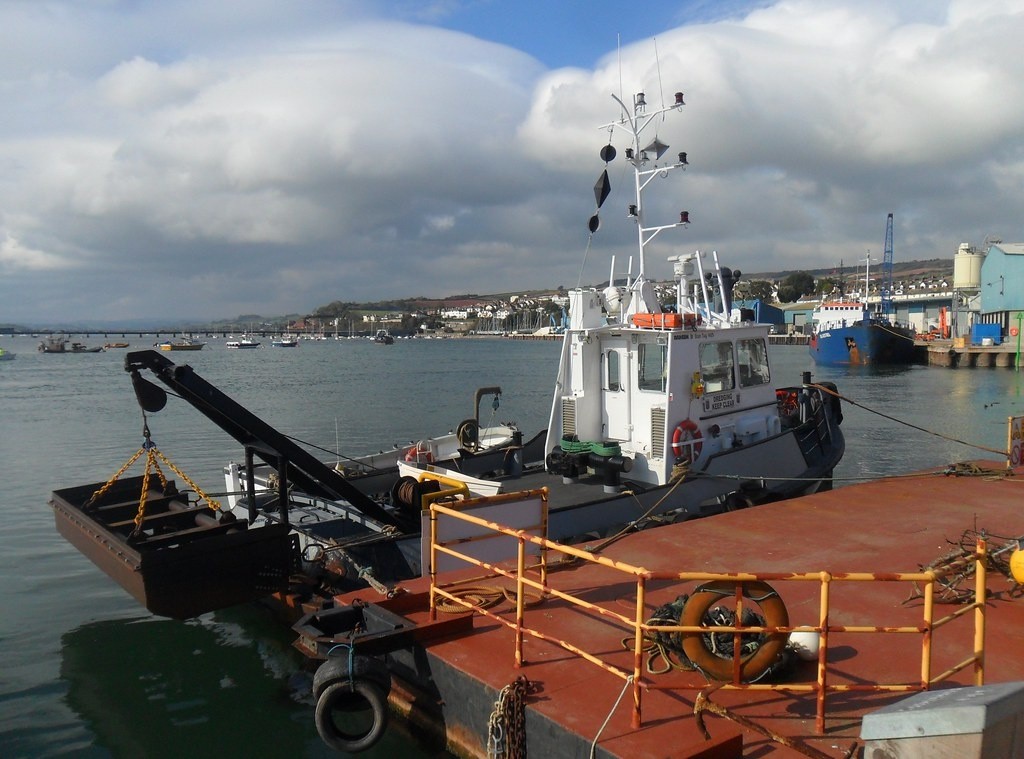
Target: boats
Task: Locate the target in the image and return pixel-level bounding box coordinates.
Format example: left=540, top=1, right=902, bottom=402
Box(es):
left=0, top=349, right=16, bottom=360
left=160, top=332, right=206, bottom=350
left=271, top=336, right=298, bottom=347
left=104, top=342, right=128, bottom=347
left=225, top=335, right=260, bottom=348
left=223, top=34, right=845, bottom=621
left=38, top=335, right=103, bottom=352
left=809, top=213, right=915, bottom=366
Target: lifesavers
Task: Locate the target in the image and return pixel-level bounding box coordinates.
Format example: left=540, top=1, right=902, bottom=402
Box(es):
left=678, top=580, right=791, bottom=686
left=310, top=652, right=394, bottom=755
left=1010, top=328, right=1018, bottom=336
left=782, top=398, right=798, bottom=415
left=404, top=446, right=435, bottom=463
left=671, top=420, right=705, bottom=464
